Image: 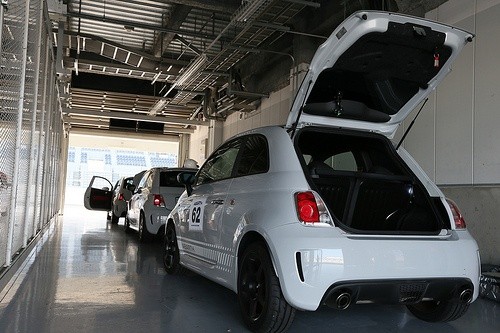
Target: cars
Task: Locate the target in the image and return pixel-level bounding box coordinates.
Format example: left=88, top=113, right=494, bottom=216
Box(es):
left=125, top=167, right=199, bottom=240
left=84, top=175, right=134, bottom=224
left=164, top=9, right=481, bottom=333
